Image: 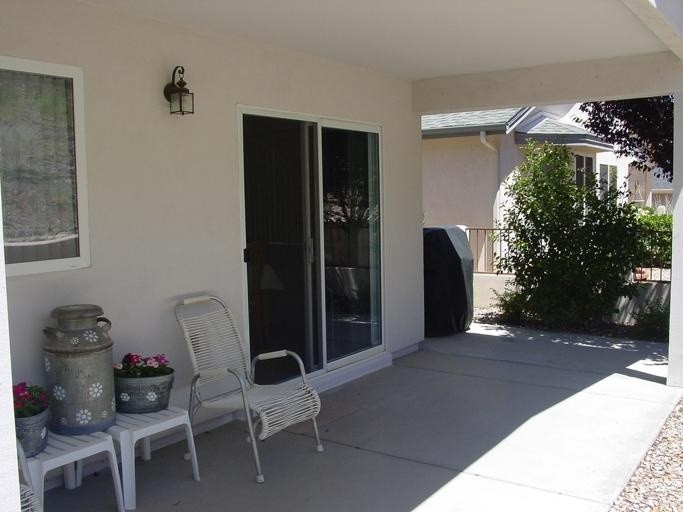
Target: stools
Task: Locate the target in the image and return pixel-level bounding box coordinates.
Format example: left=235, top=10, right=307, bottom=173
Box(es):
left=26, top=404, right=201, bottom=512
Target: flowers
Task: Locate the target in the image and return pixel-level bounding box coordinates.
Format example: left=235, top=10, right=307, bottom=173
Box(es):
left=12, top=382, right=50, bottom=418
left=114, top=352, right=174, bottom=378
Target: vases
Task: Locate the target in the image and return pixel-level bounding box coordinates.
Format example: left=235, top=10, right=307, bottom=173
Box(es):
left=114, top=367, right=175, bottom=414
left=16, top=406, right=50, bottom=458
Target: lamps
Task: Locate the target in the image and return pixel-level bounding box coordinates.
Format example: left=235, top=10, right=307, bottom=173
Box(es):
left=163, top=66, right=194, bottom=115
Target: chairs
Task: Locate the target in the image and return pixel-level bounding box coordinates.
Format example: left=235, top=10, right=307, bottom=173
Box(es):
left=174, top=295, right=323, bottom=483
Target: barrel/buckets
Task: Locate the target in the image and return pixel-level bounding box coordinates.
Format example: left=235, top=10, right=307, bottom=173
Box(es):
left=40, top=304, right=120, bottom=436
